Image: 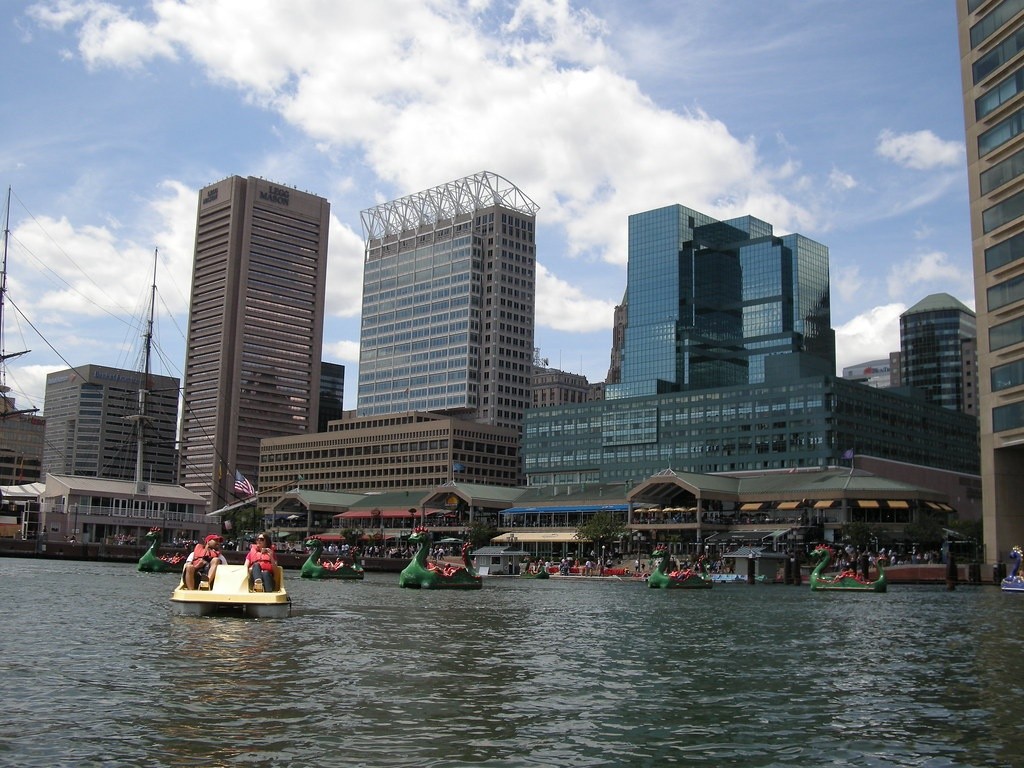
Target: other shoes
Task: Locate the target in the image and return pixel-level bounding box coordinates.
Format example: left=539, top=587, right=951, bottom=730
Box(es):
left=202, top=575, right=209, bottom=581
left=254, top=578, right=262, bottom=583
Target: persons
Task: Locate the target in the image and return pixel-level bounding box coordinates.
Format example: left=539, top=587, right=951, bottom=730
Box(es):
left=174, top=538, right=237, bottom=552
left=326, top=544, right=446, bottom=559
left=183, top=534, right=224, bottom=590
left=333, top=558, right=342, bottom=569
left=669, top=566, right=691, bottom=581
left=635, top=559, right=639, bottom=571
left=585, top=558, right=592, bottom=578
left=545, top=559, right=551, bottom=573
left=427, top=559, right=456, bottom=577
left=244, top=532, right=277, bottom=592
left=159, top=552, right=185, bottom=564
left=837, top=546, right=930, bottom=566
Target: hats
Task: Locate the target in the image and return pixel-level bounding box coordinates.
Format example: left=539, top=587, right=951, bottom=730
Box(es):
left=205, top=534, right=224, bottom=543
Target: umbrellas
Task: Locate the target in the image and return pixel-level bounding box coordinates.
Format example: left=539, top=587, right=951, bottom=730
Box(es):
left=287, top=514, right=299, bottom=528
left=634, top=507, right=706, bottom=512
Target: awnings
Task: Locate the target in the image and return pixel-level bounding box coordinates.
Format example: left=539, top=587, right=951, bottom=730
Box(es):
left=776, top=502, right=801, bottom=509
left=925, top=502, right=953, bottom=511
left=332, top=508, right=444, bottom=529
left=498, top=503, right=641, bottom=527
left=813, top=500, right=834, bottom=509
left=251, top=532, right=291, bottom=537
left=858, top=500, right=879, bottom=508
left=490, top=532, right=596, bottom=559
left=740, top=503, right=763, bottom=511
left=887, top=500, right=910, bottom=509
left=305, top=535, right=395, bottom=540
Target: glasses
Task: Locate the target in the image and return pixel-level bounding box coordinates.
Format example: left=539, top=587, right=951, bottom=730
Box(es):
left=256, top=538, right=265, bottom=541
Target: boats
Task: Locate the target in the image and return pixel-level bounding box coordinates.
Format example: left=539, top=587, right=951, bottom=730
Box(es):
left=169, top=550, right=293, bottom=619
left=1000, top=546, right=1024, bottom=592
left=808, top=544, right=888, bottom=592
left=399, top=525, right=482, bottom=590
left=299, top=535, right=364, bottom=579
left=137, top=525, right=186, bottom=574
left=520, top=557, right=549, bottom=579
left=647, top=544, right=714, bottom=590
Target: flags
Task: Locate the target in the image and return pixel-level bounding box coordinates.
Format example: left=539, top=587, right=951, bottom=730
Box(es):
left=234, top=468, right=255, bottom=496
left=840, top=447, right=854, bottom=460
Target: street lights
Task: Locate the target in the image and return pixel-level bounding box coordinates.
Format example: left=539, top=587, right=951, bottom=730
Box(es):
left=705, top=545, right=709, bottom=557
left=602, top=545, right=606, bottom=566
